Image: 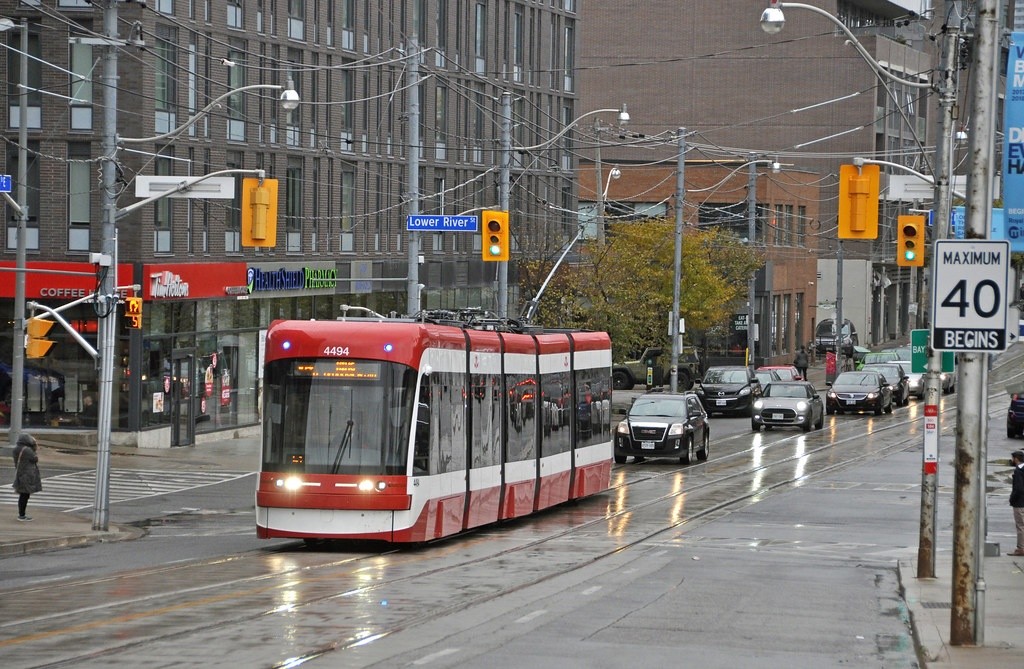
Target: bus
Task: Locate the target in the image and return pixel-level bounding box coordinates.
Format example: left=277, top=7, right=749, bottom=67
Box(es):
left=254, top=306, right=615, bottom=546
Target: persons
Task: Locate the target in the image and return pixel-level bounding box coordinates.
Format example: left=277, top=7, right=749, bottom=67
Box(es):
left=13, top=433, right=42, bottom=522
left=1007, top=450, right=1024, bottom=556
left=795, top=344, right=809, bottom=381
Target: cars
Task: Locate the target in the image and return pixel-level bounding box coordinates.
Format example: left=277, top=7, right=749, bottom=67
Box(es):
left=825, top=370, right=893, bottom=416
left=815, top=317, right=859, bottom=358
left=752, top=365, right=805, bottom=392
left=693, top=365, right=762, bottom=417
left=1007, top=381, right=1024, bottom=439
left=751, top=379, right=824, bottom=433
left=854, top=340, right=960, bottom=407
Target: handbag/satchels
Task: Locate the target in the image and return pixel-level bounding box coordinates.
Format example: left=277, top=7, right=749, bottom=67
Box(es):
left=12, top=470, right=18, bottom=487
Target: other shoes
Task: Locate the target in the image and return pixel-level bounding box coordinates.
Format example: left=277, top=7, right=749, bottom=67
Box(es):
left=17, top=514, right=34, bottom=521
left=1007, top=548, right=1024, bottom=556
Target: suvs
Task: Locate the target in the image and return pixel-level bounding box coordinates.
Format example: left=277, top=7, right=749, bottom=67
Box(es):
left=614, top=388, right=710, bottom=465
left=612, top=345, right=707, bottom=392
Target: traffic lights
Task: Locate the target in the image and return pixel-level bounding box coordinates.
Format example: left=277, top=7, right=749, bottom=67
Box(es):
left=897, top=215, right=925, bottom=266
left=482, top=210, right=510, bottom=262
left=124, top=297, right=142, bottom=330
left=27, top=317, right=59, bottom=359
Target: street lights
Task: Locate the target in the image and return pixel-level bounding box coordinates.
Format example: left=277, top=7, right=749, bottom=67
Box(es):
left=90, top=71, right=302, bottom=533
left=0, top=15, right=28, bottom=443
left=498, top=92, right=632, bottom=325
left=761, top=0, right=960, bottom=577
left=593, top=133, right=623, bottom=248
left=669, top=125, right=781, bottom=392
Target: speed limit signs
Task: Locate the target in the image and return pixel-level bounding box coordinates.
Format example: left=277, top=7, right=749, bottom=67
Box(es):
left=931, top=240, right=1010, bottom=353
